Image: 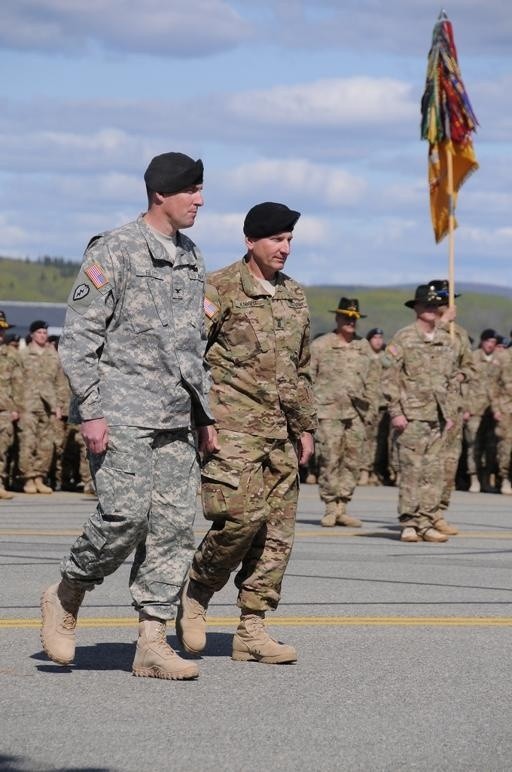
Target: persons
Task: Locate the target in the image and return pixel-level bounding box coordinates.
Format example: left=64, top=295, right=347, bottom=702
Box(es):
left=381, top=284, right=459, bottom=542
left=0, top=310, right=100, bottom=500
left=305, top=298, right=382, bottom=529
left=427, top=279, right=473, bottom=534
left=305, top=329, right=512, bottom=495
left=173, top=202, right=318, bottom=666
left=40, top=151, right=221, bottom=679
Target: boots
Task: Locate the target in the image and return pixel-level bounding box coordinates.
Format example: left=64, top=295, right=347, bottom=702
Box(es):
left=21, top=475, right=54, bottom=496
left=468, top=473, right=483, bottom=494
left=0, top=482, right=14, bottom=501
left=36, top=571, right=87, bottom=666
left=129, top=604, right=202, bottom=681
left=320, top=498, right=364, bottom=529
left=230, top=606, right=299, bottom=665
left=174, top=568, right=210, bottom=657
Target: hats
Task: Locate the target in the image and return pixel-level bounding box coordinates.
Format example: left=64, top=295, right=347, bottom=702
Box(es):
left=325, top=295, right=369, bottom=320
left=241, top=200, right=302, bottom=242
left=141, top=150, right=206, bottom=195
left=403, top=278, right=463, bottom=307
left=27, top=320, right=50, bottom=334
left=480, top=325, right=500, bottom=343
left=0, top=311, right=16, bottom=331
left=365, top=327, right=384, bottom=341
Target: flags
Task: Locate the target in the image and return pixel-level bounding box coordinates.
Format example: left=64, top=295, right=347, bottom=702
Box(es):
left=421, top=16, right=480, bottom=245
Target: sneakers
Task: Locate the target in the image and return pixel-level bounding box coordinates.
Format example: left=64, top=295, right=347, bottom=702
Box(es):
left=305, top=464, right=317, bottom=485
left=498, top=477, right=512, bottom=496
left=399, top=517, right=460, bottom=544
left=356, top=468, right=402, bottom=488
